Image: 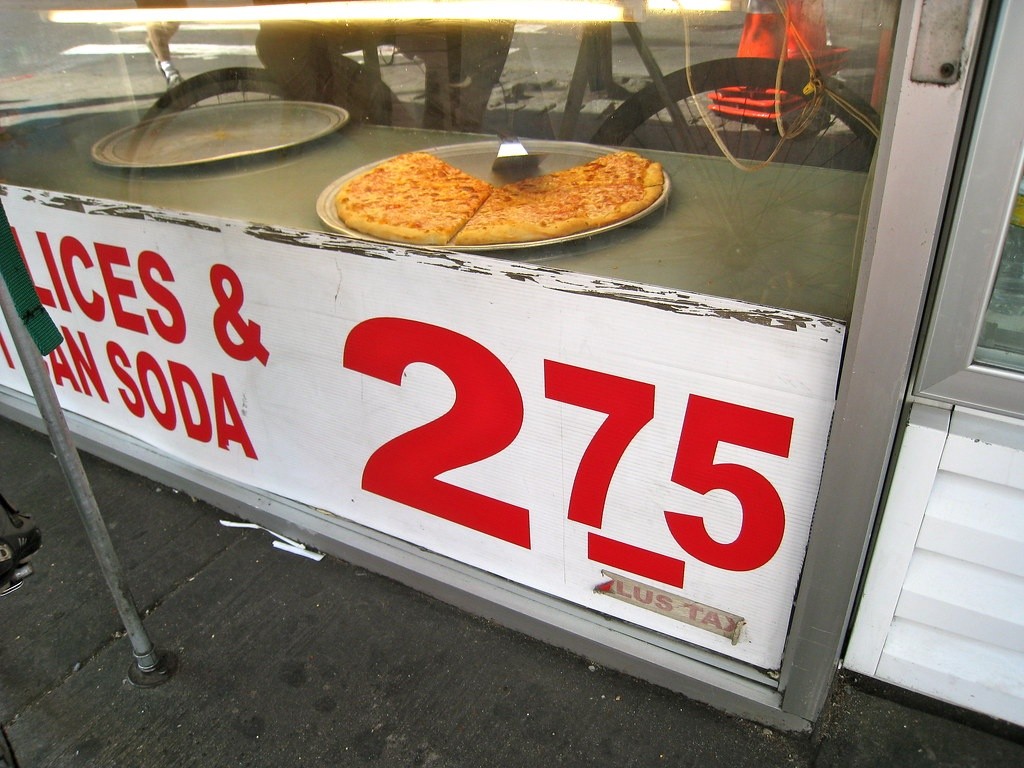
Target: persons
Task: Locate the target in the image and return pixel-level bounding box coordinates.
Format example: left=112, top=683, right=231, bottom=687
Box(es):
left=135, top=0, right=516, bottom=132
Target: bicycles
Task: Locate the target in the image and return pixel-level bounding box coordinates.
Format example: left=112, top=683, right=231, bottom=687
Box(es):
left=127, top=0, right=883, bottom=178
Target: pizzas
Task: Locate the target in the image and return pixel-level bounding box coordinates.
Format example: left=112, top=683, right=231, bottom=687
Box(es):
left=455, top=152, right=665, bottom=246
left=335, top=152, right=494, bottom=246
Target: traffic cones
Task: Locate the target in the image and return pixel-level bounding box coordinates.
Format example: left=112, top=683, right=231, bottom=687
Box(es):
left=707, top=1, right=852, bottom=122
